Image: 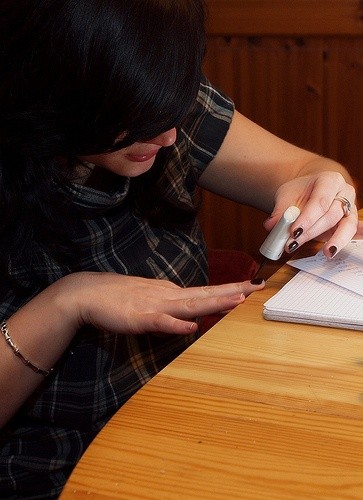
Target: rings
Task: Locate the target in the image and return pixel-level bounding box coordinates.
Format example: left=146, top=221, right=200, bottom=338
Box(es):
left=334, top=196, right=351, bottom=217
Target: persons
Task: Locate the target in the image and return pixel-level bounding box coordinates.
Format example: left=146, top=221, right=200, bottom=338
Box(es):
left=0, top=0, right=358, bottom=500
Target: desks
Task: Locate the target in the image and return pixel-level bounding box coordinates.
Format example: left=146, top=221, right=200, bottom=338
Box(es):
left=57, top=208, right=362, bottom=500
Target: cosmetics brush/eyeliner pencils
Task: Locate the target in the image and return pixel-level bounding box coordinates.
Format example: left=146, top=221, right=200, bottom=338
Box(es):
left=254, top=206, right=300, bottom=280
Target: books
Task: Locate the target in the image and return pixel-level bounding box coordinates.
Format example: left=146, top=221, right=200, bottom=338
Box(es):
left=263, top=240, right=363, bottom=330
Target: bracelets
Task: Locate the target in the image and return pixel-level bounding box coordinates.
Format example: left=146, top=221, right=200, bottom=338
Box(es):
left=0, top=320, right=54, bottom=375
